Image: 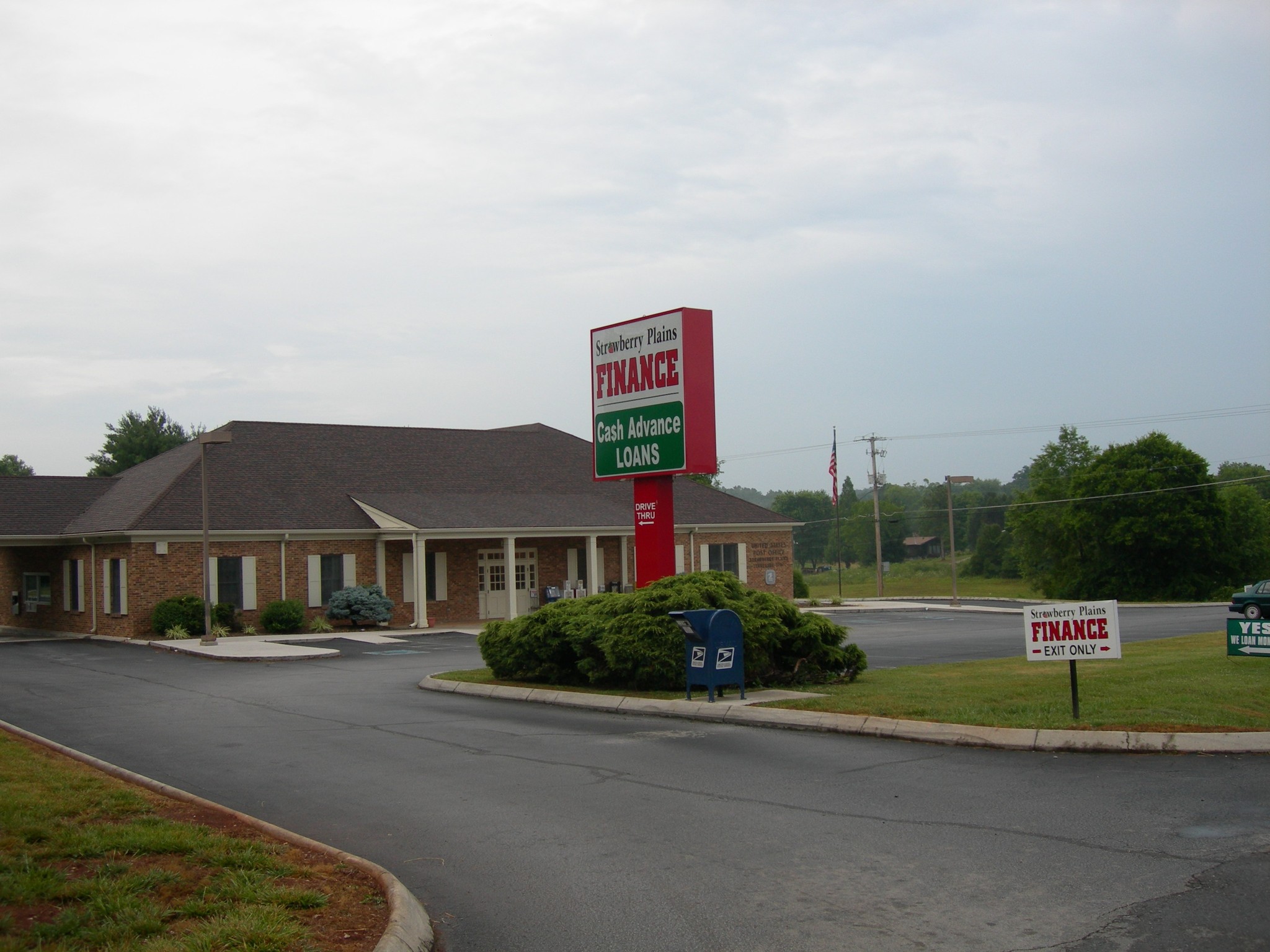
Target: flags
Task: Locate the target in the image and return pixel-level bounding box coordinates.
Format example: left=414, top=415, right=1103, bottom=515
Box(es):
left=828, top=429, right=838, bottom=506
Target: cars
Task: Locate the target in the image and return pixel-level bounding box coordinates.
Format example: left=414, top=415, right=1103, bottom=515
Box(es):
left=1227, top=579, right=1270, bottom=619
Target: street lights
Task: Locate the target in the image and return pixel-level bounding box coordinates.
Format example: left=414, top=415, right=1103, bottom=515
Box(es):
left=944, top=475, right=975, bottom=607
left=198, top=431, right=232, bottom=644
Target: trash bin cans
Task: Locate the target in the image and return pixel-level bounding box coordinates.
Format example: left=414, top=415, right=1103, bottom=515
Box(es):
left=666, top=608, right=746, bottom=703
